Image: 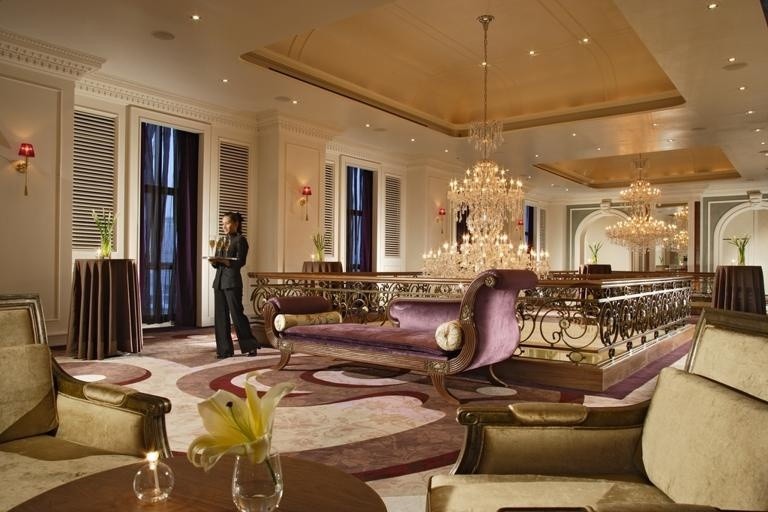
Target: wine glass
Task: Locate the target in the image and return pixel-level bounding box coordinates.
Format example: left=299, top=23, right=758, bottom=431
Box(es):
left=208, top=238, right=230, bottom=257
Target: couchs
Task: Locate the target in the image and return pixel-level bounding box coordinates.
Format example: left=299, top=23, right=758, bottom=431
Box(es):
left=262, top=269, right=540, bottom=407
left=422, top=306, right=767, bottom=512
left=0, top=292, right=174, bottom=512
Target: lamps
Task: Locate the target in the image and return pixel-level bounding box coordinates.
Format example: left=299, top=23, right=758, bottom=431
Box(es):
left=516, top=215, right=524, bottom=241
left=419, top=13, right=550, bottom=280
left=297, top=184, right=312, bottom=221
left=433, top=203, right=447, bottom=234
left=602, top=153, right=677, bottom=257
left=664, top=202, right=689, bottom=254
left=12, top=142, right=36, bottom=197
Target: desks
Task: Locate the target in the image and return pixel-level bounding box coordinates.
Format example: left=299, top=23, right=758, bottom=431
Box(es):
left=4, top=452, right=388, bottom=512
left=581, top=264, right=613, bottom=278
left=301, top=262, right=346, bottom=304
left=709, top=265, right=767, bottom=317
left=66, top=259, right=147, bottom=360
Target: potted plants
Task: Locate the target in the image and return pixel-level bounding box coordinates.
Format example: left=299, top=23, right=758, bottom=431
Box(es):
left=89, top=204, right=123, bottom=259
left=311, top=231, right=331, bottom=261
left=588, top=240, right=604, bottom=262
left=725, top=232, right=755, bottom=265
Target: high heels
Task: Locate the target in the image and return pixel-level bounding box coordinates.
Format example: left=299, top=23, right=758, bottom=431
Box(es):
left=247, top=346, right=261, bottom=357
left=215, top=353, right=233, bottom=359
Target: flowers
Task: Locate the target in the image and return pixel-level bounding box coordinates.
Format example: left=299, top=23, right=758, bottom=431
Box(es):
left=186, top=371, right=298, bottom=487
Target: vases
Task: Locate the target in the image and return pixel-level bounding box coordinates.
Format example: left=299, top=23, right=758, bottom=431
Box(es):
left=231, top=447, right=285, bottom=512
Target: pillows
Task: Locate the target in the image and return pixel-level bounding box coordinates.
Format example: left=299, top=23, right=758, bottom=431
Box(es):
left=272, top=311, right=343, bottom=332
left=0, top=342, right=62, bottom=445
left=628, top=365, right=767, bottom=512
left=434, top=319, right=465, bottom=352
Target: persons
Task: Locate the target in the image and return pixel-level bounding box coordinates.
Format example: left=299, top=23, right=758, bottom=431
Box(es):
left=208, top=212, right=261, bottom=359
